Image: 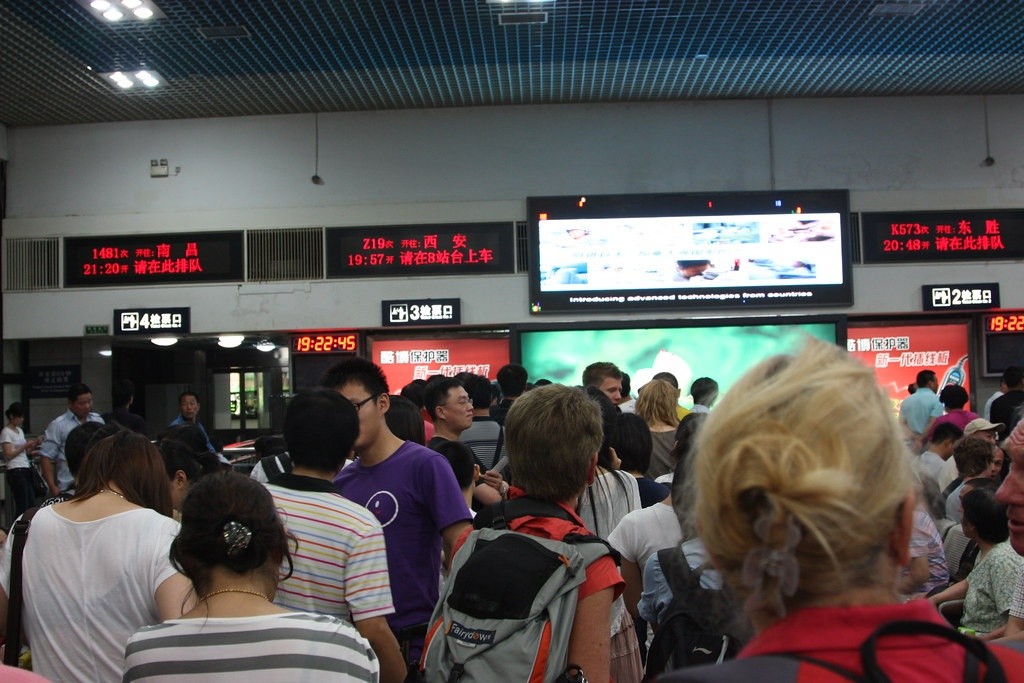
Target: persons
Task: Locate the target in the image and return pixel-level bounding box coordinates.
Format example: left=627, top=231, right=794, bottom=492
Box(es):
left=149, top=423, right=220, bottom=523
left=894, top=365, right=1024, bottom=641
left=170, top=391, right=215, bottom=453
left=99, top=379, right=145, bottom=431
left=259, top=387, right=408, bottom=683
left=449, top=384, right=627, bottom=683
left=39, top=383, right=105, bottom=496
left=385, top=364, right=552, bottom=596
left=571, top=362, right=724, bottom=670
left=0, top=399, right=43, bottom=516
left=691, top=335, right=1024, bottom=683
left=0, top=421, right=199, bottom=683
left=318, top=356, right=473, bottom=683
left=122, top=470, right=379, bottom=683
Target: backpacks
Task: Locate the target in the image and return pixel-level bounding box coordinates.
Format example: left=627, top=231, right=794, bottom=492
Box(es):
left=640, top=550, right=745, bottom=683
left=417, top=506, right=609, bottom=683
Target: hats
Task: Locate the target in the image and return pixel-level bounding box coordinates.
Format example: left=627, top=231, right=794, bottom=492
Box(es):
left=963, top=417, right=1006, bottom=439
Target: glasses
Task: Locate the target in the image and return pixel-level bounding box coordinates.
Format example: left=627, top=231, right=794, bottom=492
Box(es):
left=352, top=393, right=380, bottom=413
left=441, top=397, right=473, bottom=410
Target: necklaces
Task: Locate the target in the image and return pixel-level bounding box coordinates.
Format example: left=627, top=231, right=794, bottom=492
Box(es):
left=100, top=490, right=123, bottom=499
left=199, top=588, right=268, bottom=602
